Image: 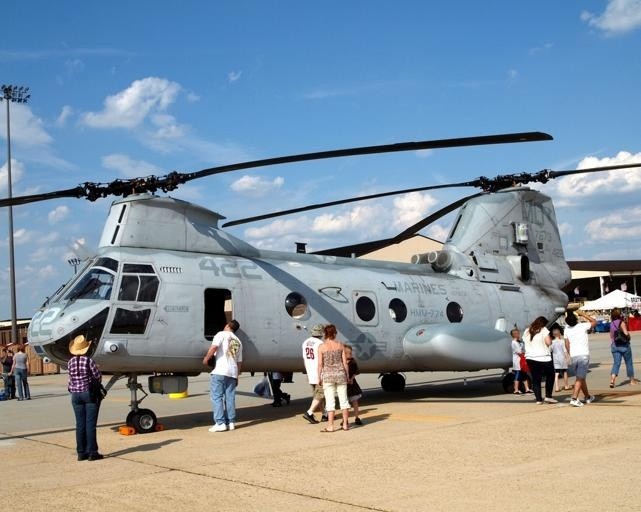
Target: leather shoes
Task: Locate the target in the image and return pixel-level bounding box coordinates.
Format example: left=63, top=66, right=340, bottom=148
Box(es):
left=286, top=395, right=290, bottom=405
left=89, top=453, right=104, bottom=461
left=78, top=454, right=89, bottom=460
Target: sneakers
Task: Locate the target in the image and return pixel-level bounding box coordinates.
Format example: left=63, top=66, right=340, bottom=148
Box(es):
left=569, top=398, right=584, bottom=407
left=355, top=418, right=362, bottom=425
left=564, top=384, right=572, bottom=389
left=555, top=388, right=561, bottom=391
left=229, top=422, right=235, bottom=430
left=537, top=402, right=543, bottom=404
left=209, top=422, right=227, bottom=432
left=321, top=415, right=337, bottom=422
left=524, top=390, right=534, bottom=393
left=583, top=395, right=595, bottom=404
left=513, top=390, right=522, bottom=394
left=340, top=419, right=348, bottom=426
left=303, top=412, right=319, bottom=423
left=544, top=397, right=558, bottom=403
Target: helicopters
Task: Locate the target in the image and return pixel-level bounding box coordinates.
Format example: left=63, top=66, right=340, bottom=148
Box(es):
left=1, top=132, right=641, bottom=432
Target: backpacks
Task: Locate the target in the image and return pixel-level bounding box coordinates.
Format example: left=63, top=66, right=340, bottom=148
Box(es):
left=613, top=319, right=630, bottom=347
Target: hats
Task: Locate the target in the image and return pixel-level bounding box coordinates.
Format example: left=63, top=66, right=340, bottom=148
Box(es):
left=311, top=323, right=324, bottom=336
left=69, top=335, right=92, bottom=355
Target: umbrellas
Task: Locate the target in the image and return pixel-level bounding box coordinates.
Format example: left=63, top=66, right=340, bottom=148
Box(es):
left=579, top=287, right=641, bottom=311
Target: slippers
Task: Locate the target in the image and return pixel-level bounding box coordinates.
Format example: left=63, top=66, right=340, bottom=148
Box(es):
left=340, top=426, right=348, bottom=431
left=320, top=427, right=334, bottom=432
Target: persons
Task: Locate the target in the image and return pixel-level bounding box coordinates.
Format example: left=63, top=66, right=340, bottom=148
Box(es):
left=549, top=327, right=573, bottom=392
left=522, top=317, right=558, bottom=403
left=509, top=329, right=533, bottom=395
left=318, top=324, right=351, bottom=433
left=0, top=348, right=15, bottom=400
left=67, top=334, right=105, bottom=462
left=608, top=308, right=640, bottom=389
left=8, top=345, right=31, bottom=401
left=202, top=319, right=242, bottom=432
left=565, top=310, right=597, bottom=406
left=343, top=345, right=362, bottom=427
left=302, top=323, right=327, bottom=425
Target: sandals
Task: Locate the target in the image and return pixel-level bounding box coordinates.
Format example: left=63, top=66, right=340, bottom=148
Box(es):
left=609, top=383, right=614, bottom=388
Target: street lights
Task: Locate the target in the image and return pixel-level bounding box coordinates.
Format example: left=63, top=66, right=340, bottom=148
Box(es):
left=0, top=83, right=32, bottom=342
left=64, top=252, right=84, bottom=277
